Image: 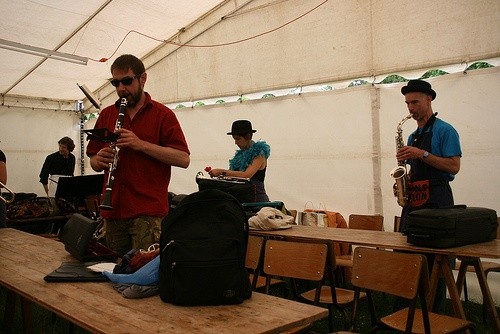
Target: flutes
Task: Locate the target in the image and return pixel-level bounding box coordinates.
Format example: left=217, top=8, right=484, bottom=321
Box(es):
left=98, top=95, right=129, bottom=211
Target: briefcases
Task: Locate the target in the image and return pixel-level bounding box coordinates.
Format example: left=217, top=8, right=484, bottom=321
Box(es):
left=197, top=175, right=255, bottom=204
left=58, top=213, right=119, bottom=262
left=406, top=206, right=498, bottom=249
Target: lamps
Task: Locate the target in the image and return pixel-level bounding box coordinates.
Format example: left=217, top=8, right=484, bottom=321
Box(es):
left=0, top=39, right=87, bottom=64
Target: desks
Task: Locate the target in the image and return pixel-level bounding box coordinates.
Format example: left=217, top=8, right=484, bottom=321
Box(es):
left=0, top=224, right=331, bottom=334
left=249, top=225, right=500, bottom=334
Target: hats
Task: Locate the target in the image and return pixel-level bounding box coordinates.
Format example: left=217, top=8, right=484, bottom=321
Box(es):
left=227, top=120, right=257, bottom=134
left=401, top=80, right=436, bottom=101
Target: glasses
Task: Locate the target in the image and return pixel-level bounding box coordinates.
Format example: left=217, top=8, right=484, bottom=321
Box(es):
left=111, top=75, right=140, bottom=87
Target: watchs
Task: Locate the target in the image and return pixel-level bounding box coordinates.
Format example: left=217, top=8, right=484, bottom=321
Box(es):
left=423, top=151, right=429, bottom=162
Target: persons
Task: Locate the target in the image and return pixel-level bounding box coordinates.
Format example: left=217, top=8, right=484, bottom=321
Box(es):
left=396, top=79, right=461, bottom=314
left=39, top=137, right=75, bottom=215
left=0, top=150, right=7, bottom=228
left=208, top=120, right=270, bottom=203
left=86, top=54, right=190, bottom=256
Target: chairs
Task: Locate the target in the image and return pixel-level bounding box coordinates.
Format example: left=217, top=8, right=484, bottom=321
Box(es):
left=245, top=211, right=500, bottom=334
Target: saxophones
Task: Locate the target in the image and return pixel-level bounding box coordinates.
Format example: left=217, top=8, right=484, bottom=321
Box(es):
left=389, top=112, right=414, bottom=207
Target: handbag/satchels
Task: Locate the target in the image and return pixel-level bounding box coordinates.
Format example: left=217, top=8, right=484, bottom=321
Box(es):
left=248, top=206, right=294, bottom=230
left=302, top=201, right=328, bottom=228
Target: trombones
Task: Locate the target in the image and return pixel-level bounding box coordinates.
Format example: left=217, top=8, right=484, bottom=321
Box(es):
left=0, top=182, right=15, bottom=204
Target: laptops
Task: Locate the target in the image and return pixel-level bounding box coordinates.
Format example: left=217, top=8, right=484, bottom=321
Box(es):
left=43, top=261, right=109, bottom=282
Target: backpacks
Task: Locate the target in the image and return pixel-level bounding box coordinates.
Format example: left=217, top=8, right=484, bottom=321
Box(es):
left=159, top=189, right=253, bottom=306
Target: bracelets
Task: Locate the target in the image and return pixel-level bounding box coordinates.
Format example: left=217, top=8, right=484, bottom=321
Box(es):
left=221, top=169, right=227, bottom=176
